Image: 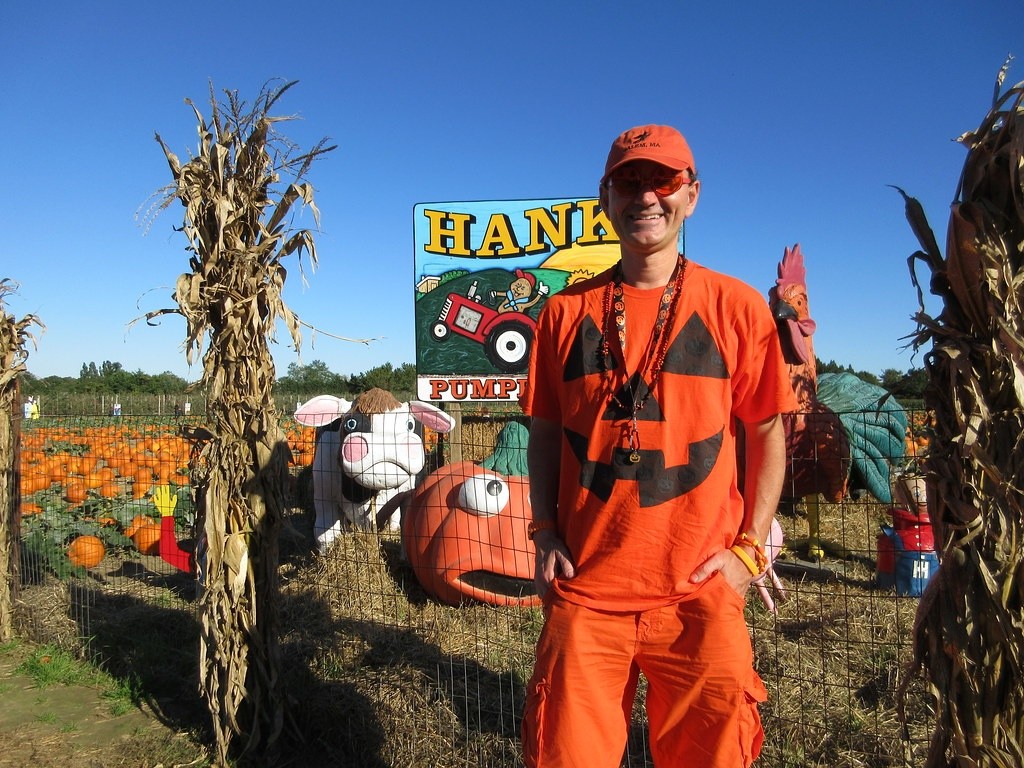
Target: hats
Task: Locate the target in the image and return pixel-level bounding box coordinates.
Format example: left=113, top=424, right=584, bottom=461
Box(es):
left=600, top=124, right=697, bottom=182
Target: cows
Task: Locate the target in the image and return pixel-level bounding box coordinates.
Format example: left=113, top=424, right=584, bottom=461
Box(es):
left=293, top=387, right=456, bottom=557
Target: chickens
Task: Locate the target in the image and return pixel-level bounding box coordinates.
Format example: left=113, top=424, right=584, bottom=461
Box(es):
left=736, top=243, right=909, bottom=564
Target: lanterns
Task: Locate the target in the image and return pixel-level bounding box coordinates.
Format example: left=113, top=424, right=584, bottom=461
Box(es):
left=406, top=420, right=544, bottom=608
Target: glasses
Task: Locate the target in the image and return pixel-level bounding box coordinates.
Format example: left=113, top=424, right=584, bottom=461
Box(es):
left=604, top=176, right=692, bottom=195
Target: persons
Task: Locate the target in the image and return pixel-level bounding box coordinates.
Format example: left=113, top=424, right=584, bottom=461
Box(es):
left=876, top=470, right=941, bottom=598
left=477, top=406, right=489, bottom=418
left=174, top=405, right=184, bottom=426
left=517, top=125, right=803, bottom=768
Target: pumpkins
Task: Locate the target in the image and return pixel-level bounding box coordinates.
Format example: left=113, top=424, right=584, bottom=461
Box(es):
left=19, top=422, right=317, bottom=568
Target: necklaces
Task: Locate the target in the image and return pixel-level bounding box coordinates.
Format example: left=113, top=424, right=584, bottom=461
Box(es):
left=614, top=253, right=681, bottom=463
left=601, top=253, right=688, bottom=411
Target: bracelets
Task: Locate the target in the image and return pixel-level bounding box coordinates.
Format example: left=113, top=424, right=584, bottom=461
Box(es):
left=527, top=520, right=556, bottom=540
left=736, top=533, right=768, bottom=574
left=730, top=545, right=760, bottom=577
